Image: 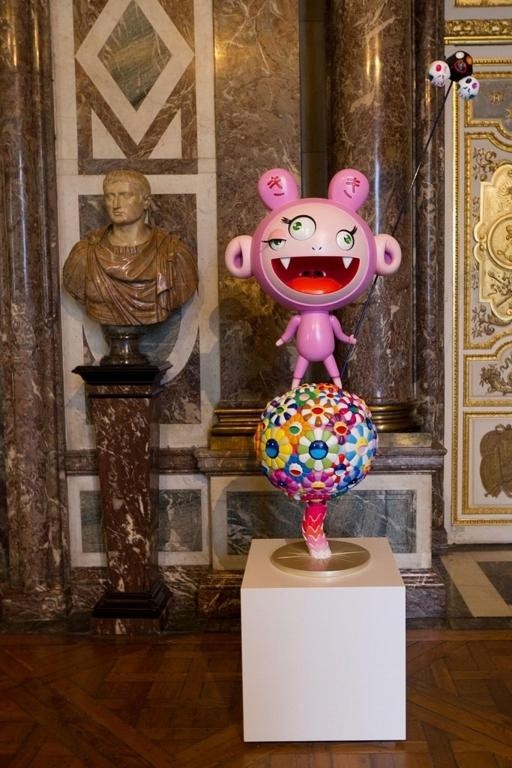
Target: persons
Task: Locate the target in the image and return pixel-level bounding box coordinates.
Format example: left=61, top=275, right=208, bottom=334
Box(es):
left=56, top=164, right=201, bottom=328
